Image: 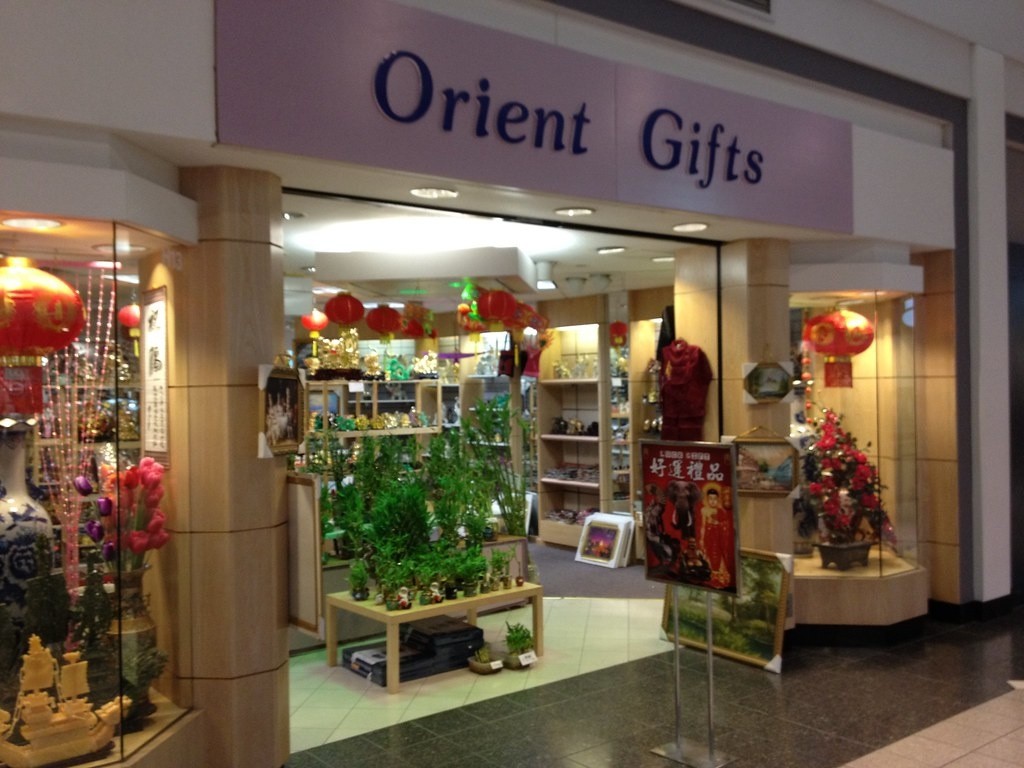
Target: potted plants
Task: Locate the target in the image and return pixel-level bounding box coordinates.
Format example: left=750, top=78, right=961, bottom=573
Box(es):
left=468, top=645, right=505, bottom=674
left=346, top=539, right=541, bottom=612
left=491, top=621, right=538, bottom=670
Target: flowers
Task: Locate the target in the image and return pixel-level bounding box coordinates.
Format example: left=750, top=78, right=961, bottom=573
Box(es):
left=791, top=401, right=904, bottom=557
left=74, top=454, right=170, bottom=570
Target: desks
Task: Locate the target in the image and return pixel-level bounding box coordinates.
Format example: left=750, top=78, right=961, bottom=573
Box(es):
left=326, top=574, right=543, bottom=695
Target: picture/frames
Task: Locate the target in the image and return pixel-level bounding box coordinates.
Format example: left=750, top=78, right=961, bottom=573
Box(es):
left=742, top=360, right=793, bottom=404
left=574, top=512, right=637, bottom=569
left=659, top=547, right=793, bottom=674
left=721, top=435, right=800, bottom=499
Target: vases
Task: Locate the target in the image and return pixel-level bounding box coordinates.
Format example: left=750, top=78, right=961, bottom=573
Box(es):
left=815, top=541, right=872, bottom=570
left=0, top=414, right=54, bottom=635
left=110, top=571, right=160, bottom=718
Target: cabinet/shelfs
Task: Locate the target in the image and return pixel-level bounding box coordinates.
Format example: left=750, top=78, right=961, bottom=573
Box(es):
left=538, top=325, right=613, bottom=548
left=307, top=378, right=442, bottom=467
left=459, top=330, right=523, bottom=474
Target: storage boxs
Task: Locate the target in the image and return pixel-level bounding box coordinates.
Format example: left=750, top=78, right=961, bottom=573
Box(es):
left=398, top=614, right=484, bottom=660
left=342, top=638, right=489, bottom=686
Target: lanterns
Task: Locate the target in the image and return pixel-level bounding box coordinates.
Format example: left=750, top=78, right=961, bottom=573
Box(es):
left=365, top=304, right=438, bottom=345
left=802, top=304, right=873, bottom=387
left=611, top=321, right=628, bottom=360
left=0, top=256, right=88, bottom=415
left=301, top=292, right=365, bottom=356
left=461, top=290, right=534, bottom=367
left=118, top=301, right=142, bottom=357
